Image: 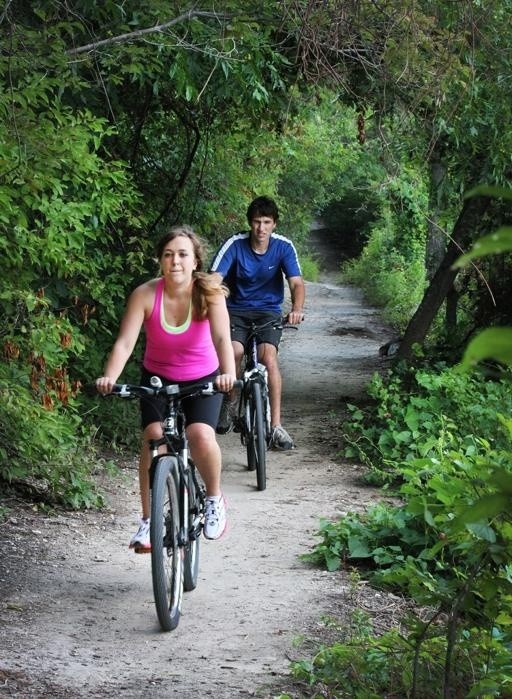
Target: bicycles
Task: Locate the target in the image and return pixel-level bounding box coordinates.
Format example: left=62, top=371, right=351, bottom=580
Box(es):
left=223, top=312, right=307, bottom=492
left=95, top=374, right=244, bottom=633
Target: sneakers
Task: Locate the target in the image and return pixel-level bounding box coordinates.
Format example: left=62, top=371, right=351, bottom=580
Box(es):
left=269, top=422, right=294, bottom=450
left=216, top=393, right=238, bottom=435
left=128, top=514, right=151, bottom=552
left=203, top=492, right=227, bottom=540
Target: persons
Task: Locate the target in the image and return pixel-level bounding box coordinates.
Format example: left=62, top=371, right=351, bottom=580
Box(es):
left=95, top=227, right=237, bottom=553
left=206, top=196, right=305, bottom=451
